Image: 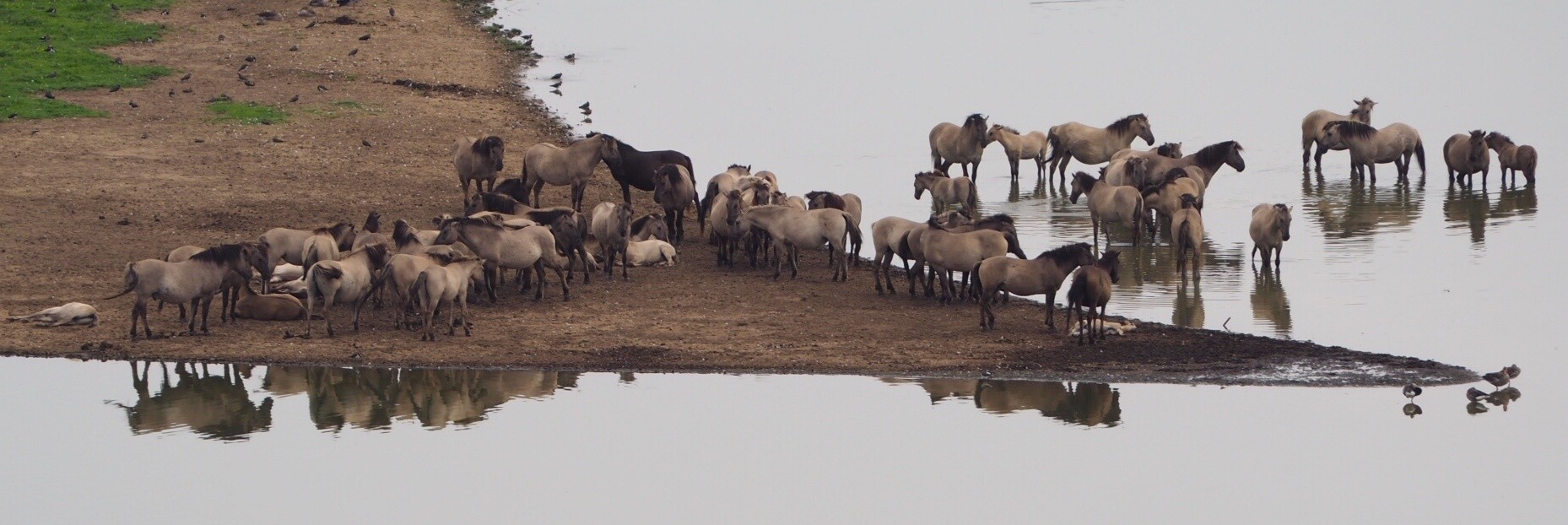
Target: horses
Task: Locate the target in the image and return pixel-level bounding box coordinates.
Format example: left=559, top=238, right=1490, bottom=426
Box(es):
left=453, top=136, right=506, bottom=215
left=103, top=359, right=581, bottom=438
left=871, top=209, right=1136, bottom=346
left=881, top=376, right=1126, bottom=428
left=586, top=131, right=704, bottom=236
left=102, top=178, right=604, bottom=339
left=591, top=202, right=632, bottom=281
left=699, top=164, right=863, bottom=284
left=521, top=133, right=624, bottom=214
left=1171, top=267, right=1293, bottom=338
left=1249, top=203, right=1293, bottom=270
left=913, top=113, right=1246, bottom=284
left=653, top=164, right=695, bottom=242
left=592, top=214, right=678, bottom=269
left=1301, top=98, right=1538, bottom=195
left=8, top=302, right=101, bottom=328
